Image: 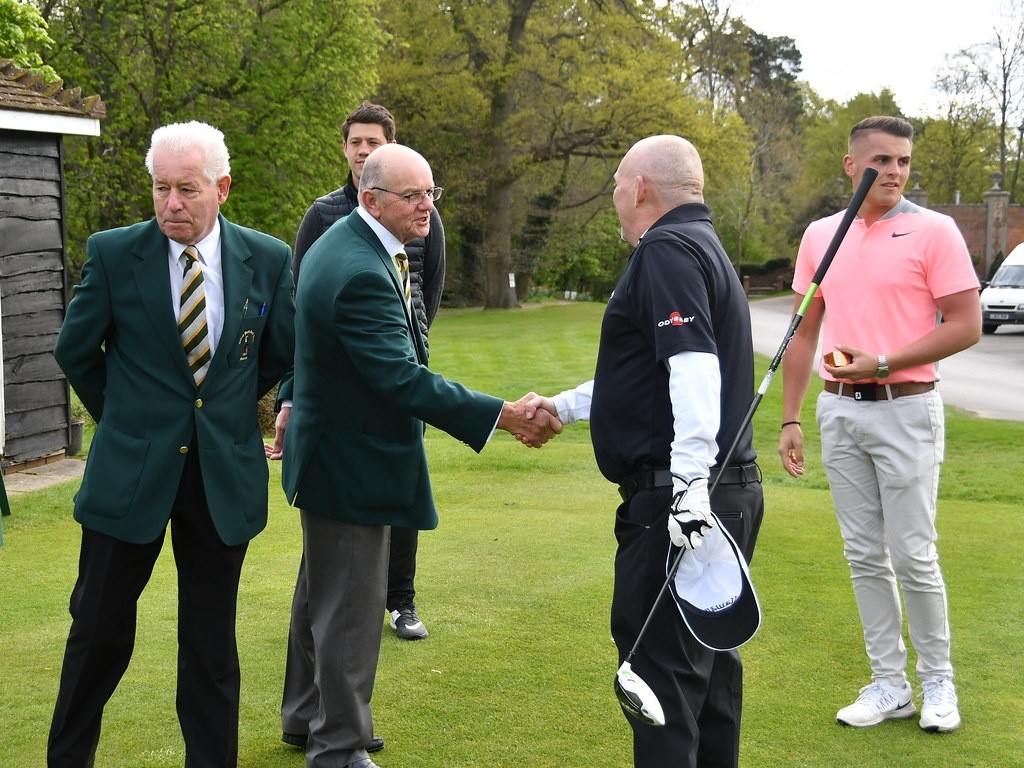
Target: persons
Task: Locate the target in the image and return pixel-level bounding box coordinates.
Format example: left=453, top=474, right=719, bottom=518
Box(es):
left=779, top=115, right=982, bottom=730
left=282, top=143, right=563, bottom=768
left=292, top=102, right=445, bottom=641
left=46, top=119, right=298, bottom=768
left=516, top=136, right=764, bottom=768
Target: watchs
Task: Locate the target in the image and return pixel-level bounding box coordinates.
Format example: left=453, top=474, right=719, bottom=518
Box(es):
left=874, top=355, right=889, bottom=379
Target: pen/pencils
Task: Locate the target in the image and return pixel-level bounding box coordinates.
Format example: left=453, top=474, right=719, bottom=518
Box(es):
left=261, top=302, right=267, bottom=317
left=242, top=300, right=249, bottom=318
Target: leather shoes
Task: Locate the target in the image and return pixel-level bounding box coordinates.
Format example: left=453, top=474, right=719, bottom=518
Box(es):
left=282, top=732, right=384, bottom=768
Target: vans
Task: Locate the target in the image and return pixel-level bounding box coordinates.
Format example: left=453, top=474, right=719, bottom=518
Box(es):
left=978, top=241, right=1024, bottom=334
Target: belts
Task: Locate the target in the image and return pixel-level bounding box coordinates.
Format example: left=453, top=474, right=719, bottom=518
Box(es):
left=824, top=379, right=934, bottom=401
left=618, top=463, right=760, bottom=501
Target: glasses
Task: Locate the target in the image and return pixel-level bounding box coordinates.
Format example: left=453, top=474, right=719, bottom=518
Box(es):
left=371, top=185, right=444, bottom=204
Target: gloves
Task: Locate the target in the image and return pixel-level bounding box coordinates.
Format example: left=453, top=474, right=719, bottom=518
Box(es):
left=667, top=475, right=713, bottom=549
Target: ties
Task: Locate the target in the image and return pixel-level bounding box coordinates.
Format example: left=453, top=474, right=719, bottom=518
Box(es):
left=177, top=246, right=212, bottom=387
left=395, top=253, right=411, bottom=323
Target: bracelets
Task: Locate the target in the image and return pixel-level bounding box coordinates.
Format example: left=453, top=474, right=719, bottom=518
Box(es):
left=781, top=422, right=801, bottom=429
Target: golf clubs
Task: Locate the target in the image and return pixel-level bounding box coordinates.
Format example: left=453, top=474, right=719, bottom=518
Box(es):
left=613, top=167, right=879, bottom=726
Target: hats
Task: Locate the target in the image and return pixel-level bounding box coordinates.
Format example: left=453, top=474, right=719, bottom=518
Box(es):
left=666, top=511, right=762, bottom=652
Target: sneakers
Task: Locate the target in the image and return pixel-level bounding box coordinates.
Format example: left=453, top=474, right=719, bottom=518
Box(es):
left=390, top=602, right=428, bottom=640
left=915, top=679, right=960, bottom=734
left=836, top=680, right=916, bottom=727
